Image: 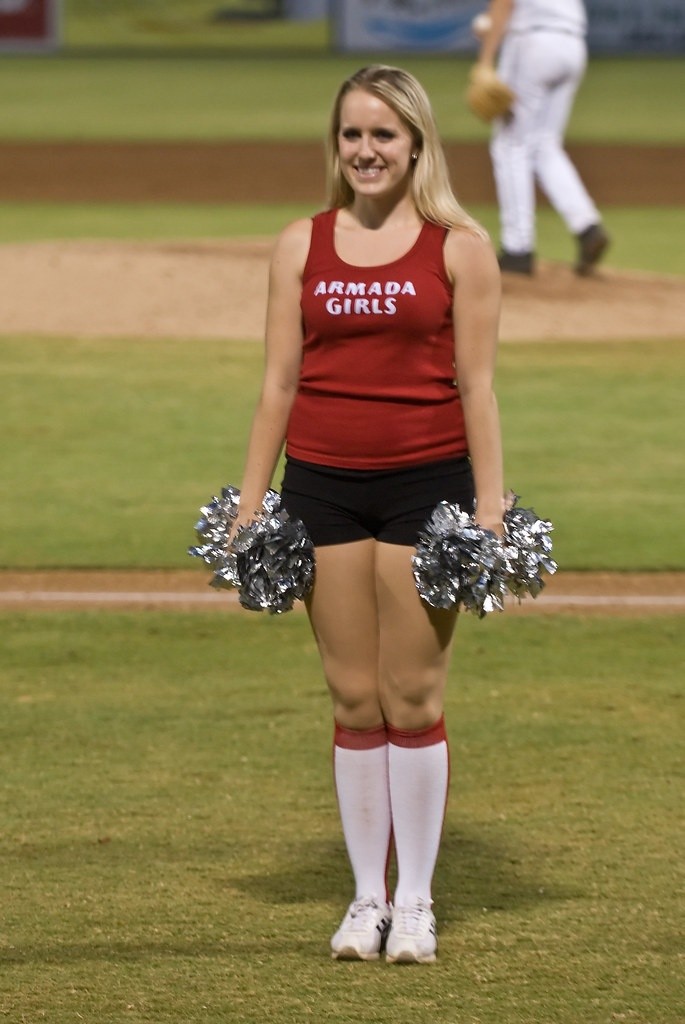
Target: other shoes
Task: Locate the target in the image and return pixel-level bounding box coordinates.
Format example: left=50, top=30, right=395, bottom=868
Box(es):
left=495, top=250, right=534, bottom=275
left=573, top=227, right=610, bottom=278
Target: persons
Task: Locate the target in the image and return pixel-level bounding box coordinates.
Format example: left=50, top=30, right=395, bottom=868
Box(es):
left=227, top=66, right=502, bottom=964
left=466, top=0, right=610, bottom=278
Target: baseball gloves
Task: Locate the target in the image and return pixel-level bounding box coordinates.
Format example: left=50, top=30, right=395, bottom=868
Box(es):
left=466, top=64, right=516, bottom=125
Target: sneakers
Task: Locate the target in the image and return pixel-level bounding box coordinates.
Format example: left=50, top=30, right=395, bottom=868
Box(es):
left=330, top=892, right=391, bottom=962
left=385, top=895, right=438, bottom=964
